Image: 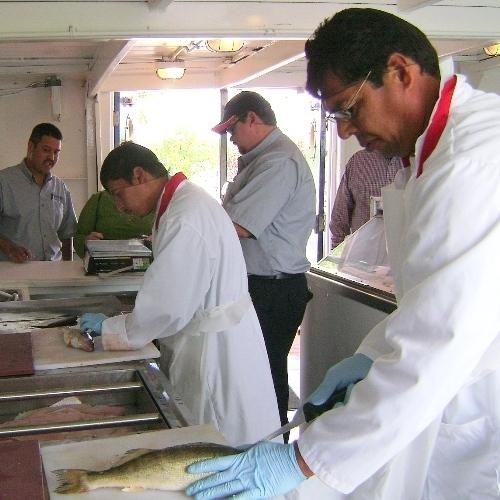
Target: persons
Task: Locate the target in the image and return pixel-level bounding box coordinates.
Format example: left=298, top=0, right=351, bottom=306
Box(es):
left=328, top=147, right=405, bottom=251
left=0, top=122, right=77, bottom=263
left=212, top=90, right=317, bottom=443
left=76, top=140, right=285, bottom=448
left=185, top=7, right=499, bottom=500
left=73, top=190, right=155, bottom=259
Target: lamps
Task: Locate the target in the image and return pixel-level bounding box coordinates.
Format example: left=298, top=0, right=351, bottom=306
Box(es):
left=154, top=59, right=187, bottom=82
left=205, top=39, right=246, bottom=55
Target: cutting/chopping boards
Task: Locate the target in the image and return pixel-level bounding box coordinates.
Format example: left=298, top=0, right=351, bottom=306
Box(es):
left=0, top=332, right=33, bottom=376
left=0, top=439, right=44, bottom=499
left=31, top=325, right=161, bottom=371
left=40, top=423, right=233, bottom=499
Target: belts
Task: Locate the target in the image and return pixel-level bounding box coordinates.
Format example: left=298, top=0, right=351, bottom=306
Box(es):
left=257, top=271, right=302, bottom=283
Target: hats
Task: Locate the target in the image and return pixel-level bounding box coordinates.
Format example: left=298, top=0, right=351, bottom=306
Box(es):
left=211, top=91, right=272, bottom=135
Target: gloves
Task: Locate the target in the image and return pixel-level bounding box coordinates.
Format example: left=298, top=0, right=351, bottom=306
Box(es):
left=184, top=439, right=308, bottom=500
left=310, top=353, right=374, bottom=409
left=79, top=312, right=110, bottom=337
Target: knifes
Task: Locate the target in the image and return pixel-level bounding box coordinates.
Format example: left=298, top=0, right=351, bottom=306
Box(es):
left=243, top=378, right=364, bottom=453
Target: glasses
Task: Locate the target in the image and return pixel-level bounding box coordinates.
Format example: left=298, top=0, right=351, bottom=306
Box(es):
left=324, top=70, right=372, bottom=124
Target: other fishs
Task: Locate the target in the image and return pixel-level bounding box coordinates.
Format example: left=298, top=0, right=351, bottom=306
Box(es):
left=31, top=317, right=78, bottom=328
left=51, top=443, right=247, bottom=495
left=62, top=326, right=94, bottom=352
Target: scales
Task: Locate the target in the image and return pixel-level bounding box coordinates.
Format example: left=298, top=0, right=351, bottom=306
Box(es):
left=83, top=239, right=152, bottom=275
